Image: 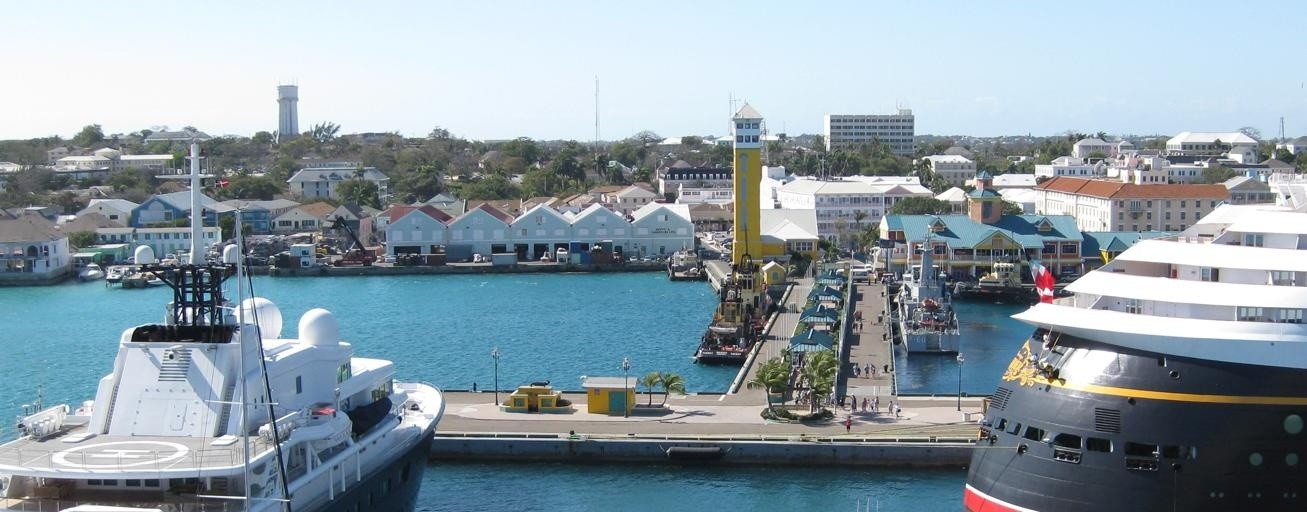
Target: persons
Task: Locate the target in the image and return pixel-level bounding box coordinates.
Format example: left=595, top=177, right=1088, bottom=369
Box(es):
left=1028, top=351, right=1040, bottom=370
left=851, top=360, right=876, bottom=379
left=849, top=394, right=899, bottom=419
left=1041, top=328, right=1057, bottom=348
left=845, top=415, right=850, bottom=431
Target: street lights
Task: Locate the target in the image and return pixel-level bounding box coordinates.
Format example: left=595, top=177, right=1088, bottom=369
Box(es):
left=956, top=353, right=964, bottom=411
left=622, top=357, right=631, bottom=419
left=492, top=347, right=500, bottom=406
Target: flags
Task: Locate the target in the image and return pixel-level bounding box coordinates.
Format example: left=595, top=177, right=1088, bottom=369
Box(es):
left=1025, top=251, right=1056, bottom=303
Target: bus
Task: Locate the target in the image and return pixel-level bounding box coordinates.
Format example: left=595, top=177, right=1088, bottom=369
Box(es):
left=836, top=269, right=872, bottom=282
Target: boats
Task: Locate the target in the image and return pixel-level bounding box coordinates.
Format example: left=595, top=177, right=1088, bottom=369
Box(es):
left=963, top=173, right=1307, bottom=512
left=669, top=249, right=768, bottom=363
left=897, top=229, right=1034, bottom=354
